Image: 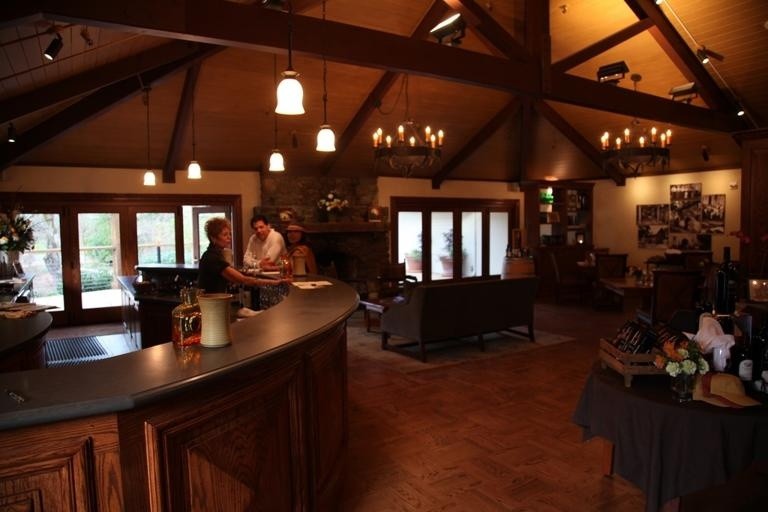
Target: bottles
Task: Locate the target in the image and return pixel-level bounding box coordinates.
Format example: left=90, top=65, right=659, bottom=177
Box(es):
left=717, top=245, right=737, bottom=308
left=506, top=240, right=531, bottom=257
left=730, top=321, right=767, bottom=380
left=710, top=269, right=732, bottom=334
left=171, top=287, right=200, bottom=348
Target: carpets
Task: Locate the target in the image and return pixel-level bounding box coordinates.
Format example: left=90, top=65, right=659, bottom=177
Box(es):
left=347, top=309, right=575, bottom=375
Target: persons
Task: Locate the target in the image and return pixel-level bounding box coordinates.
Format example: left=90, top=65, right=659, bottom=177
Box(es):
left=242, top=214, right=289, bottom=272
left=198, top=217, right=295, bottom=295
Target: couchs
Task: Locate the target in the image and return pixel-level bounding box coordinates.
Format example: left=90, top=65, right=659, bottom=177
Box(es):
left=381, top=277, right=537, bottom=363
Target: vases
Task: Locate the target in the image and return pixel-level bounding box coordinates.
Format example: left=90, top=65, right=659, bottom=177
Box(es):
left=670, top=371, right=695, bottom=402
left=323, top=207, right=337, bottom=223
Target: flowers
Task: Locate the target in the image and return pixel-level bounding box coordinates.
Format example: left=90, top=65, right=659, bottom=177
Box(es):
left=316, top=194, right=350, bottom=212
left=654, top=340, right=710, bottom=378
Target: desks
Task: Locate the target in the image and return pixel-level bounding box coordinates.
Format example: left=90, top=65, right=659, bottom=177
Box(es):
left=359, top=296, right=404, bottom=332
left=599, top=277, right=653, bottom=312
left=572, top=361, right=768, bottom=512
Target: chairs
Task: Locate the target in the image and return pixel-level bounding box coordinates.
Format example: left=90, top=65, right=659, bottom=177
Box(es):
left=376, top=262, right=418, bottom=297
left=653, top=271, right=697, bottom=312
left=595, top=254, right=627, bottom=306
